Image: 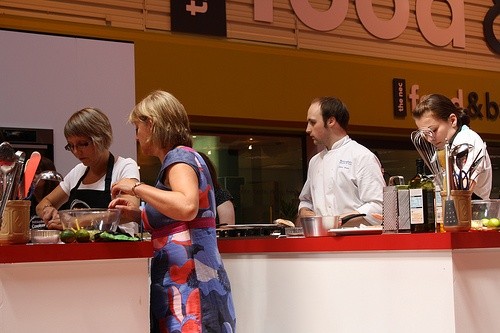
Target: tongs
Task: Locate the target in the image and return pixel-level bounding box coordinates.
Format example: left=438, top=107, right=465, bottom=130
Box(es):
left=0, top=150, right=25, bottom=229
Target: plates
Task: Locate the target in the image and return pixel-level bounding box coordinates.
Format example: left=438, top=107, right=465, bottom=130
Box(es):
left=327, top=226, right=383, bottom=235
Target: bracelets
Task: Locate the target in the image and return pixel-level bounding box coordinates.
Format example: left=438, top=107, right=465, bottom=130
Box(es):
left=131, top=183, right=141, bottom=197
left=42, top=205, right=51, bottom=210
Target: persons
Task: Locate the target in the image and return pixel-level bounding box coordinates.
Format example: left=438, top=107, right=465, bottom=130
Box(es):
left=108, top=91, right=237, bottom=333
left=298, top=97, right=386, bottom=228
left=413, top=93, right=492, bottom=199
left=36, top=107, right=141, bottom=231
left=25, top=157, right=68, bottom=230
left=197, top=152, right=235, bottom=225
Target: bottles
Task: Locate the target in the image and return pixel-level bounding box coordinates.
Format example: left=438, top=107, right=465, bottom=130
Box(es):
left=408, top=157, right=435, bottom=233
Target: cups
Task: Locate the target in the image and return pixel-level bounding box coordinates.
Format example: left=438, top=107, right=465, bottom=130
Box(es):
left=440, top=190, right=471, bottom=231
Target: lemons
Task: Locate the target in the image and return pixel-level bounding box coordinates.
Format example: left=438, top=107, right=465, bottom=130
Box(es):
left=59, top=229, right=90, bottom=243
left=480, top=217, right=500, bottom=227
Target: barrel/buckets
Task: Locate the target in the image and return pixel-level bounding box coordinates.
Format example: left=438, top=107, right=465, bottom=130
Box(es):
left=0, top=199, right=31, bottom=244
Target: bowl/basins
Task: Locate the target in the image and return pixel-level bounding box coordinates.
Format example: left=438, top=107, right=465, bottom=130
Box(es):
left=470, top=199, right=500, bottom=230
left=32, top=229, right=60, bottom=243
left=57, top=207, right=122, bottom=242
left=300, top=215, right=340, bottom=238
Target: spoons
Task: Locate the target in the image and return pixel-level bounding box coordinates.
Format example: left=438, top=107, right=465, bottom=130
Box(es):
left=456, top=144, right=468, bottom=190
left=0, top=142, right=16, bottom=208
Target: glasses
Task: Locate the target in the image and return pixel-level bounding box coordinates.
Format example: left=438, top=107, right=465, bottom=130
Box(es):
left=65, top=139, right=92, bottom=151
